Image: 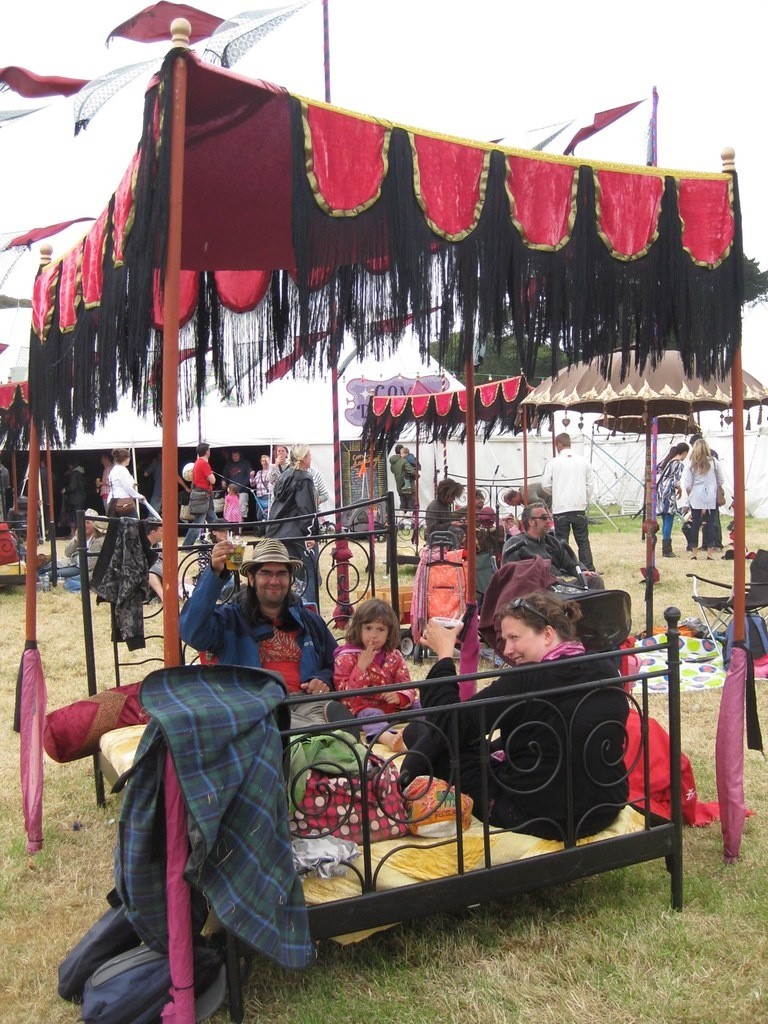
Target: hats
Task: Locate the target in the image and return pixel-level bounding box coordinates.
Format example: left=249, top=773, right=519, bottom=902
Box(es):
left=85, top=509, right=98, bottom=517
left=93, top=516, right=110, bottom=533
left=239, top=538, right=304, bottom=577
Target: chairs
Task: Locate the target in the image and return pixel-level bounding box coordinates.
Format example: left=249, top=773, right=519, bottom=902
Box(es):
left=686, top=549, right=768, bottom=660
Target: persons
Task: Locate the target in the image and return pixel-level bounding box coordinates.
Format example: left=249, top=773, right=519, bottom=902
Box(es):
left=0, top=444, right=329, bottom=604
left=389, top=445, right=422, bottom=519
left=178, top=537, right=629, bottom=842
left=542, top=433, right=595, bottom=571
left=502, top=503, right=608, bottom=595
left=425, top=479, right=552, bottom=552
left=656, top=435, right=724, bottom=560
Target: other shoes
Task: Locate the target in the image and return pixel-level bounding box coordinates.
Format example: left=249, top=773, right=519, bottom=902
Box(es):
left=322, top=700, right=372, bottom=751
left=708, top=556, right=715, bottom=560
left=691, top=556, right=698, bottom=560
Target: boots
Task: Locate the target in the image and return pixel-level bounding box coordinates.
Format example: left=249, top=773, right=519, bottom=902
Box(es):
left=662, top=539, right=680, bottom=558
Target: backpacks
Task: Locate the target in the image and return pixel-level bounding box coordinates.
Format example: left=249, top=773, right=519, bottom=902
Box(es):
left=722, top=611, right=768, bottom=678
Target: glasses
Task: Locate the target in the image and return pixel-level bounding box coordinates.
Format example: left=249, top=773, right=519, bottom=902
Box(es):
left=529, top=515, right=552, bottom=520
left=255, top=572, right=291, bottom=580
left=505, top=598, right=554, bottom=629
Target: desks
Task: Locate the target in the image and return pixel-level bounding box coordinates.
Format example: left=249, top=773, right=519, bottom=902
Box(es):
left=180, top=492, right=249, bottom=538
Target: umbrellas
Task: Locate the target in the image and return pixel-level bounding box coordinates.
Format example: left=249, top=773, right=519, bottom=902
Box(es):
left=594, top=413, right=702, bottom=541
left=517, top=347, right=768, bottom=563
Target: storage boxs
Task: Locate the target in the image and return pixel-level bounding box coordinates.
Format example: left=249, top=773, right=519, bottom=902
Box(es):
left=356, top=585, right=417, bottom=625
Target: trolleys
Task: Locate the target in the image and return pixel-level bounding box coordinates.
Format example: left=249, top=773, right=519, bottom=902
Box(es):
left=413, top=530, right=468, bottom=665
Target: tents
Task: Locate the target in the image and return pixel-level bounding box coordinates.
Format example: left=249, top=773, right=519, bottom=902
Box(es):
left=25, top=46, right=746, bottom=1024
left=0, top=377, right=82, bottom=582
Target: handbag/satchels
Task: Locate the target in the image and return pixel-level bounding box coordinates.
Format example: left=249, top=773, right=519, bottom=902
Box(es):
left=716, top=485, right=725, bottom=506
left=189, top=491, right=209, bottom=514
left=112, top=498, right=135, bottom=515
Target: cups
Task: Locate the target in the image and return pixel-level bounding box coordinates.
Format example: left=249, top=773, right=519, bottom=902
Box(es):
left=223, top=544, right=246, bottom=570
left=429, top=617, right=461, bottom=657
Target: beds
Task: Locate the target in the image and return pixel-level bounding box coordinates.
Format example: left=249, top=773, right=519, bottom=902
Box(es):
left=0, top=520, right=40, bottom=584
left=382, top=462, right=542, bottom=576
left=74, top=490, right=687, bottom=1023
